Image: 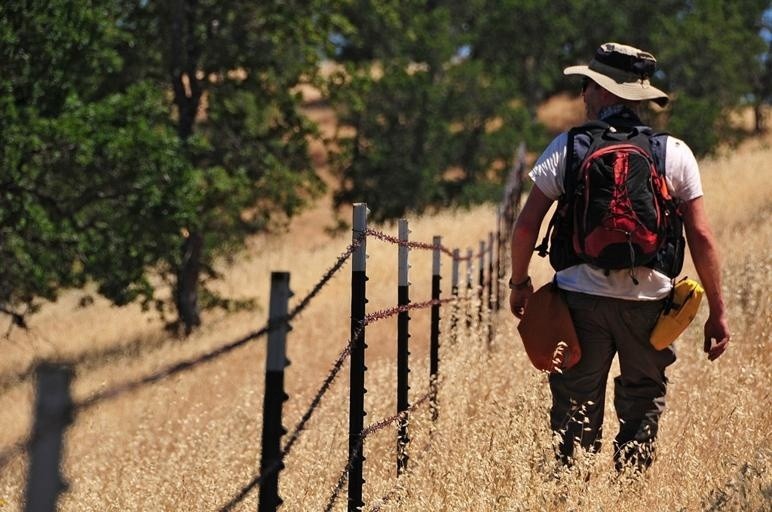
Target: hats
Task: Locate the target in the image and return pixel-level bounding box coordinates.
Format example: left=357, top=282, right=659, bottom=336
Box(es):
left=562, top=40, right=670, bottom=109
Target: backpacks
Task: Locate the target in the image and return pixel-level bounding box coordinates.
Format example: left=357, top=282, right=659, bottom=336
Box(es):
left=561, top=126, right=676, bottom=268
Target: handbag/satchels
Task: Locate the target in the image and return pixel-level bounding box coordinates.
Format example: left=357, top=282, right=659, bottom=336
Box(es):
left=515, top=280, right=581, bottom=375
left=649, top=277, right=704, bottom=352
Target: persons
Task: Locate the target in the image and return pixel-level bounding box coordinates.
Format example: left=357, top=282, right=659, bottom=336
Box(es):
left=508, top=40, right=731, bottom=486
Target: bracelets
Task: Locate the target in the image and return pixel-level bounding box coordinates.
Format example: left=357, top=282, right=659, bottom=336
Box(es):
left=508, top=276, right=532, bottom=290
